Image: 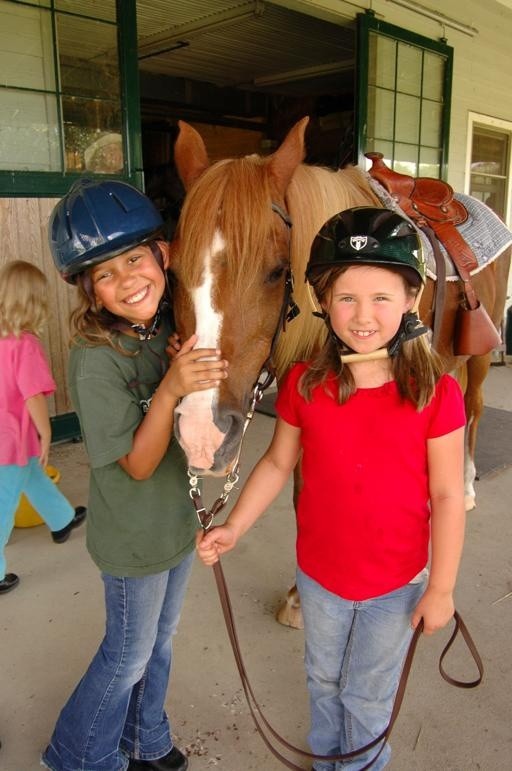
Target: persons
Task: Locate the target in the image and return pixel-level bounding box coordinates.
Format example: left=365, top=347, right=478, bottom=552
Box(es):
left=194, top=201, right=474, bottom=771
left=0, top=258, right=86, bottom=597
left=34, top=178, right=230, bottom=770
left=85, top=124, right=130, bottom=176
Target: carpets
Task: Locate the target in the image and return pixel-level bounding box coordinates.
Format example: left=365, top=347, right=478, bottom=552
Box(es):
left=249, top=390, right=512, bottom=479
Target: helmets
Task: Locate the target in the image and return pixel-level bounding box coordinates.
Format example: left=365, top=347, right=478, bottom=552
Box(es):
left=47, top=178, right=169, bottom=285
left=304, top=205, right=427, bottom=287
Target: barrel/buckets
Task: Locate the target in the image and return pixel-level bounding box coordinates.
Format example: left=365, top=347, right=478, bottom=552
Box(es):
left=16, top=469, right=59, bottom=530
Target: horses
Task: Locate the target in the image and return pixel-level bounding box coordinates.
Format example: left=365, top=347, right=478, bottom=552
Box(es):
left=169, top=115, right=512, bottom=632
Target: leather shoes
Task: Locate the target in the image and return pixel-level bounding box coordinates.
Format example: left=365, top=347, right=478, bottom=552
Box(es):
left=126, top=746, right=188, bottom=771
left=0, top=573, right=19, bottom=594
left=51, top=505, right=87, bottom=544
